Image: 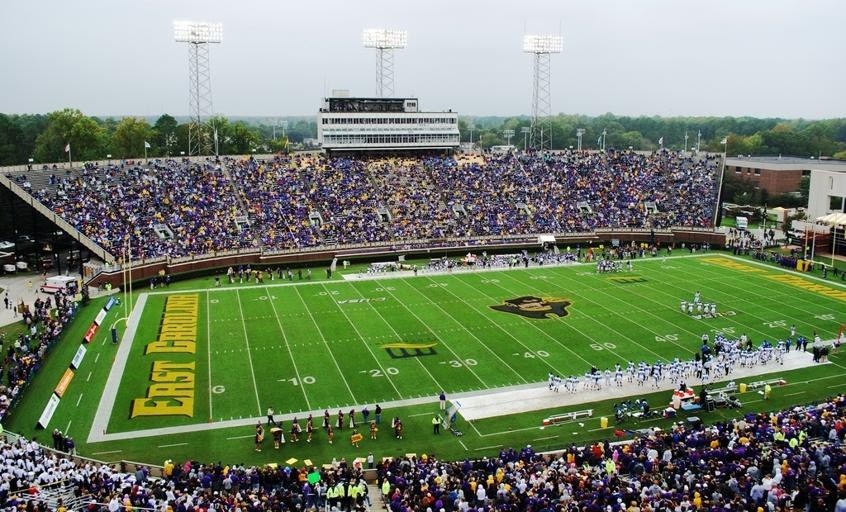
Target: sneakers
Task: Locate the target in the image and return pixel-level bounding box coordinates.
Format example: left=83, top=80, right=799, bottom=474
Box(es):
left=274, top=439, right=332, bottom=449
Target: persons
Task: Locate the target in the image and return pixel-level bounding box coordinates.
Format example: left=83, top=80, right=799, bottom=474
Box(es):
left=688, top=301, right=694, bottom=316
left=696, top=301, right=703, bottom=317
left=0, top=284, right=114, bottom=324
left=680, top=300, right=687, bottom=313
left=711, top=303, right=716, bottom=318
left=703, top=302, right=710, bottom=317
left=693, top=290, right=701, bottom=304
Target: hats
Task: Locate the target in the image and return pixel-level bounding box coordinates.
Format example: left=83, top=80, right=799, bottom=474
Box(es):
left=606, top=487, right=633, bottom=512
left=418, top=469, right=446, bottom=484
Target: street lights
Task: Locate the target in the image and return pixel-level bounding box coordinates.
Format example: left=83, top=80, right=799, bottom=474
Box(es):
left=362, top=27, right=408, bottom=96
left=172, top=21, right=223, bottom=156
left=520, top=34, right=563, bottom=150
